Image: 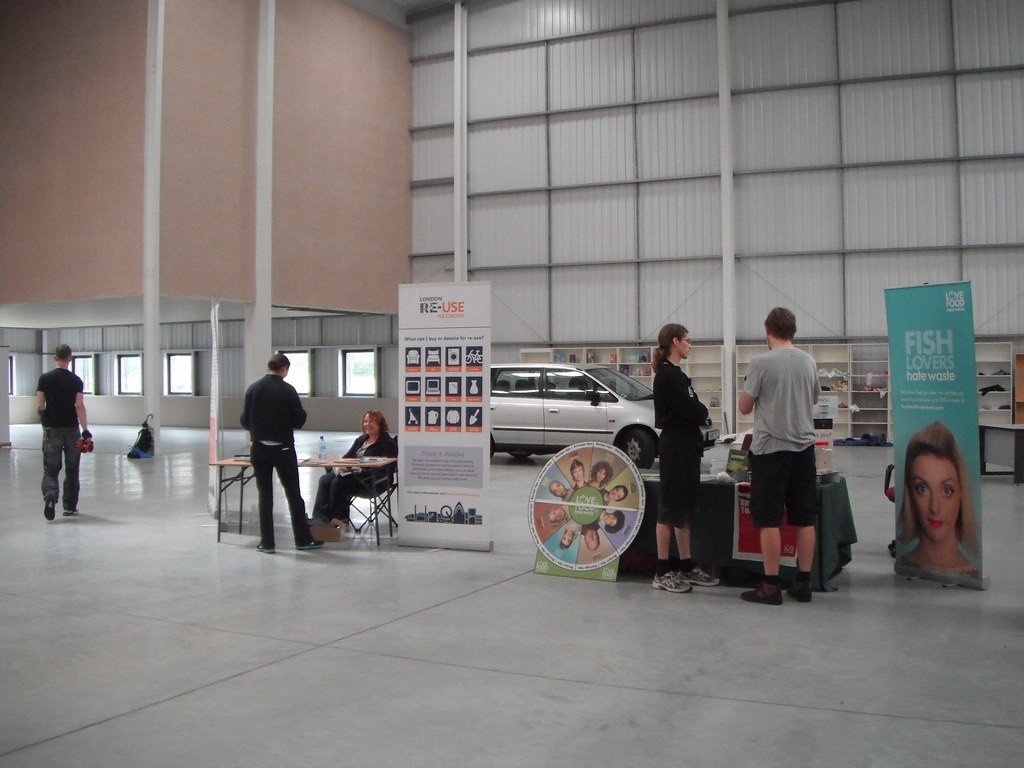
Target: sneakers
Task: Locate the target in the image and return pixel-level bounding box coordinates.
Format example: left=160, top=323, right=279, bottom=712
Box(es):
left=652, top=571, right=693, bottom=594
left=679, top=564, right=719, bottom=586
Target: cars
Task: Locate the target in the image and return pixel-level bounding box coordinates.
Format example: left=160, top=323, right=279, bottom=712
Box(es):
left=489, top=363, right=719, bottom=469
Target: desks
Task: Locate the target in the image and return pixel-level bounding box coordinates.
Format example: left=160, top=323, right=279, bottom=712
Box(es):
left=978, top=423, right=1024, bottom=486
left=209, top=455, right=398, bottom=548
left=620, top=470, right=858, bottom=591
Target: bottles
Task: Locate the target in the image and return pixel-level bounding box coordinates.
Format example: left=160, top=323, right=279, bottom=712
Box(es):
left=814, top=442, right=833, bottom=475
left=317, top=436, right=326, bottom=463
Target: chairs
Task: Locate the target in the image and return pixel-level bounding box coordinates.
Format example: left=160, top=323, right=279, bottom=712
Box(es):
left=883, top=463, right=896, bottom=559
left=322, top=435, right=399, bottom=546
left=499, top=380, right=511, bottom=391
left=515, top=379, right=531, bottom=392
left=569, top=374, right=586, bottom=389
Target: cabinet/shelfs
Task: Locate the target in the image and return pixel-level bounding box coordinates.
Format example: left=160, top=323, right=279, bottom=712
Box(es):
left=1014, top=353, right=1024, bottom=423
left=975, top=342, right=1013, bottom=423
left=517, top=344, right=723, bottom=444
left=735, top=344, right=850, bottom=446
left=850, top=340, right=890, bottom=441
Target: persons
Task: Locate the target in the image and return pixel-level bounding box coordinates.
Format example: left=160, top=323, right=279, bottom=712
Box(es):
left=559, top=518, right=582, bottom=549
left=650, top=323, right=720, bottom=593
left=549, top=481, right=574, bottom=502
left=589, top=461, right=613, bottom=490
left=313, top=410, right=399, bottom=529
left=240, top=354, right=324, bottom=554
left=541, top=504, right=572, bottom=528
left=36, top=343, right=92, bottom=521
left=570, top=459, right=588, bottom=491
left=597, top=509, right=625, bottom=534
left=599, top=486, right=628, bottom=506
left=739, top=307, right=822, bottom=605
left=581, top=521, right=601, bottom=551
left=898, top=421, right=981, bottom=578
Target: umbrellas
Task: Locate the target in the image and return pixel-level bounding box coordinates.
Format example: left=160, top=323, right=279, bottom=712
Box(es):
left=134, top=413, right=153, bottom=450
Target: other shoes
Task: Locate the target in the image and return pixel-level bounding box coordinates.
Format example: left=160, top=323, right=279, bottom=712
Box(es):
left=63, top=508, right=78, bottom=516
left=44, top=493, right=55, bottom=520
left=740, top=580, right=782, bottom=604
left=296, top=540, right=324, bottom=550
left=256, top=542, right=274, bottom=554
left=787, top=582, right=812, bottom=602
